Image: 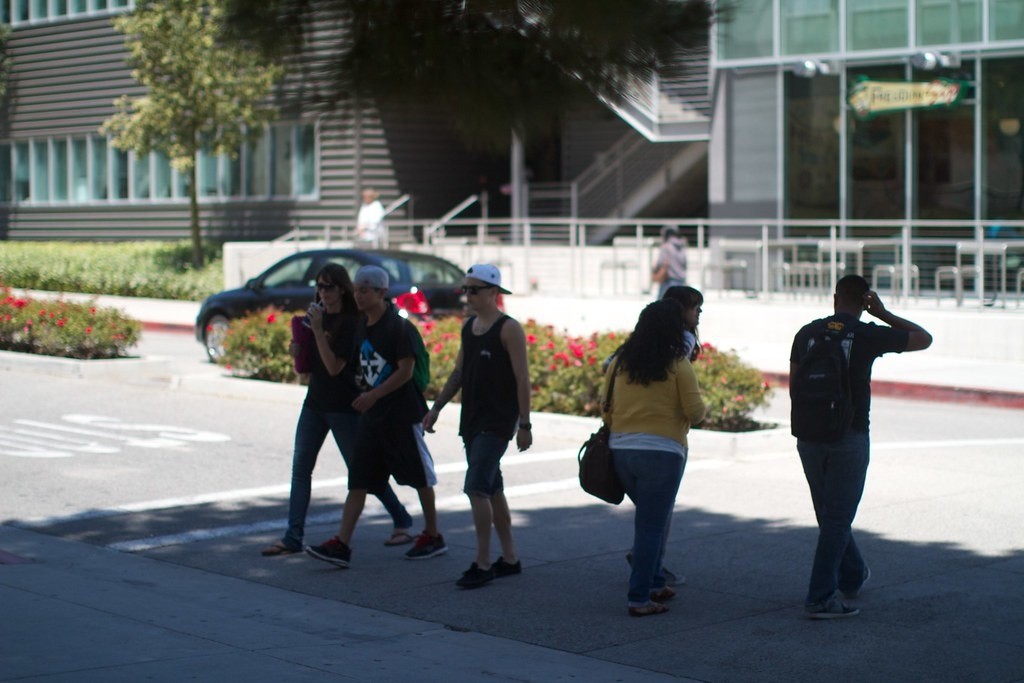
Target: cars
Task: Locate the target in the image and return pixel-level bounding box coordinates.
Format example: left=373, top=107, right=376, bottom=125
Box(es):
left=942, top=203, right=1024, bottom=292
left=194, top=246, right=473, bottom=365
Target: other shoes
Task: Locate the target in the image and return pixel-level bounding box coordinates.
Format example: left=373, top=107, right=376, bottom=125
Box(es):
left=650, top=584, right=677, bottom=599
left=628, top=601, right=669, bottom=616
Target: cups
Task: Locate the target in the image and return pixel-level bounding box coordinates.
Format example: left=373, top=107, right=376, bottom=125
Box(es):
left=301, top=302, right=324, bottom=329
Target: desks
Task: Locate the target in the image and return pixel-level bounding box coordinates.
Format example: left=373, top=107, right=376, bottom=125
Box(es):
left=816, top=238, right=902, bottom=306
left=610, top=235, right=688, bottom=297
left=954, top=239, right=1024, bottom=310
left=709, top=235, right=825, bottom=298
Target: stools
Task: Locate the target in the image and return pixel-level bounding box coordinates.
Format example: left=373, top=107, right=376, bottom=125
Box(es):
left=934, top=263, right=987, bottom=308
left=599, top=256, right=843, bottom=300
left=1015, top=266, right=1024, bottom=310
left=872, top=262, right=920, bottom=308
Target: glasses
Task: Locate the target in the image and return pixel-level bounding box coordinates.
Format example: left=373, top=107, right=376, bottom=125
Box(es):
left=461, top=284, right=494, bottom=293
left=314, top=282, right=337, bottom=293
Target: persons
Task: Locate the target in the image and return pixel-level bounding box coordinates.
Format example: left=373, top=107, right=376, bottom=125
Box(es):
left=646, top=222, right=688, bottom=303
left=355, top=189, right=389, bottom=250
left=599, top=285, right=707, bottom=617
left=419, top=264, right=532, bottom=590
left=789, top=275, right=933, bottom=617
left=261, top=264, right=449, bottom=568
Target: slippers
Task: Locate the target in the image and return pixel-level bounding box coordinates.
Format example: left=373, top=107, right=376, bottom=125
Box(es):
left=384, top=532, right=422, bottom=547
left=261, top=544, right=304, bottom=557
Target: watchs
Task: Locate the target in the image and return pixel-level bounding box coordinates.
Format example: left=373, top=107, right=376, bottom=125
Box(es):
left=519, top=423, right=531, bottom=431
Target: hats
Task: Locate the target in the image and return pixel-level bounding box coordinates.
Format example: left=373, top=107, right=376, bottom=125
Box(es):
left=466, top=263, right=512, bottom=295
left=659, top=225, right=681, bottom=240
left=354, top=265, right=389, bottom=289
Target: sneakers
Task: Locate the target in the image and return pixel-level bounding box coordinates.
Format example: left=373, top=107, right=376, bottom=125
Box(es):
left=652, top=565, right=686, bottom=586
left=305, top=535, right=353, bottom=568
left=803, top=591, right=860, bottom=617
left=455, top=561, right=497, bottom=587
left=841, top=565, right=871, bottom=597
left=403, top=530, right=449, bottom=560
left=492, top=556, right=522, bottom=578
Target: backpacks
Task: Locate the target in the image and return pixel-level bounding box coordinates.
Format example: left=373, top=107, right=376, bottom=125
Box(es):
left=790, top=343, right=850, bottom=441
left=403, top=316, right=430, bottom=393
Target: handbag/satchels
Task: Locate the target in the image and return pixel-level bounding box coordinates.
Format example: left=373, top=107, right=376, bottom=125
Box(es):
left=578, top=424, right=626, bottom=505
left=651, top=259, right=668, bottom=282
left=292, top=316, right=320, bottom=375
